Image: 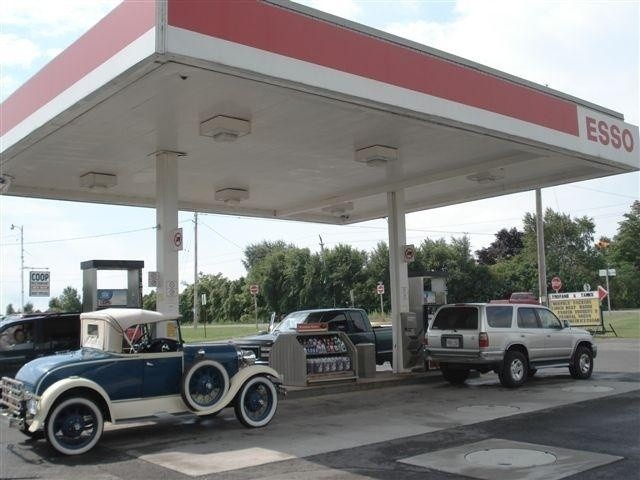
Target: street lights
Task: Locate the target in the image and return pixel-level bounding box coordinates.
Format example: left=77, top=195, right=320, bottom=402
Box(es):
left=378, top=280, right=383, bottom=313
left=9, top=225, right=50, bottom=307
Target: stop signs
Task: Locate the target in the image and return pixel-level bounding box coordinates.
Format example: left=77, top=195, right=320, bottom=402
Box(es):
left=550, top=277, right=562, bottom=292
left=377, top=285, right=384, bottom=294
left=250, top=285, right=258, bottom=294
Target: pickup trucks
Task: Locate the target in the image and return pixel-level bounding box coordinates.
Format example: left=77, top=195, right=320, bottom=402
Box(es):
left=235, top=307, right=392, bottom=368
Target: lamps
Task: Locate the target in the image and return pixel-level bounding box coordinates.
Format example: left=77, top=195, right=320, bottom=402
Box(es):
left=200, top=116, right=252, bottom=143
left=321, top=198, right=353, bottom=213
left=354, top=145, right=395, bottom=168
left=80, top=172, right=118, bottom=190
left=214, top=188, right=250, bottom=205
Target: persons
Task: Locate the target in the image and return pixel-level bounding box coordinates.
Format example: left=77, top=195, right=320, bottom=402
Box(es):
left=13, top=328, right=26, bottom=343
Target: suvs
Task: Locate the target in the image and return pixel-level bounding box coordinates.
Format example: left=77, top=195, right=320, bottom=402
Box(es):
left=423, top=302, right=597, bottom=387
